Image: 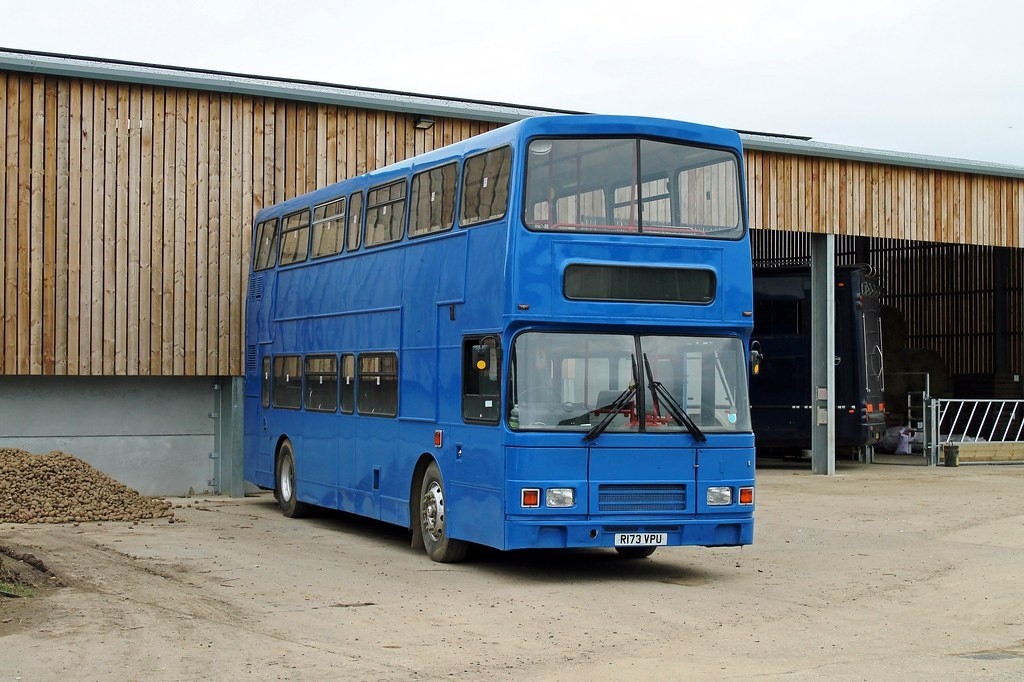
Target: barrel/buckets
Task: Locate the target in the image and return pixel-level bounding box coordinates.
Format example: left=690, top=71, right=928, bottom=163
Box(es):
left=944, top=440, right=960, bottom=466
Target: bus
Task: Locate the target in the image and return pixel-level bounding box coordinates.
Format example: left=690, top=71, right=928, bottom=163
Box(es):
left=749, top=263, right=887, bottom=462
left=242, top=114, right=764, bottom=564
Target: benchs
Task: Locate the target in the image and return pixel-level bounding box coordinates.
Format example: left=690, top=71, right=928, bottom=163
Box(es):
left=266, top=377, right=396, bottom=414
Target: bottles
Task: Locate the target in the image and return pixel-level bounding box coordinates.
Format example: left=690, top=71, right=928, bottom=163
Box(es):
left=509, top=404, right=520, bottom=428
